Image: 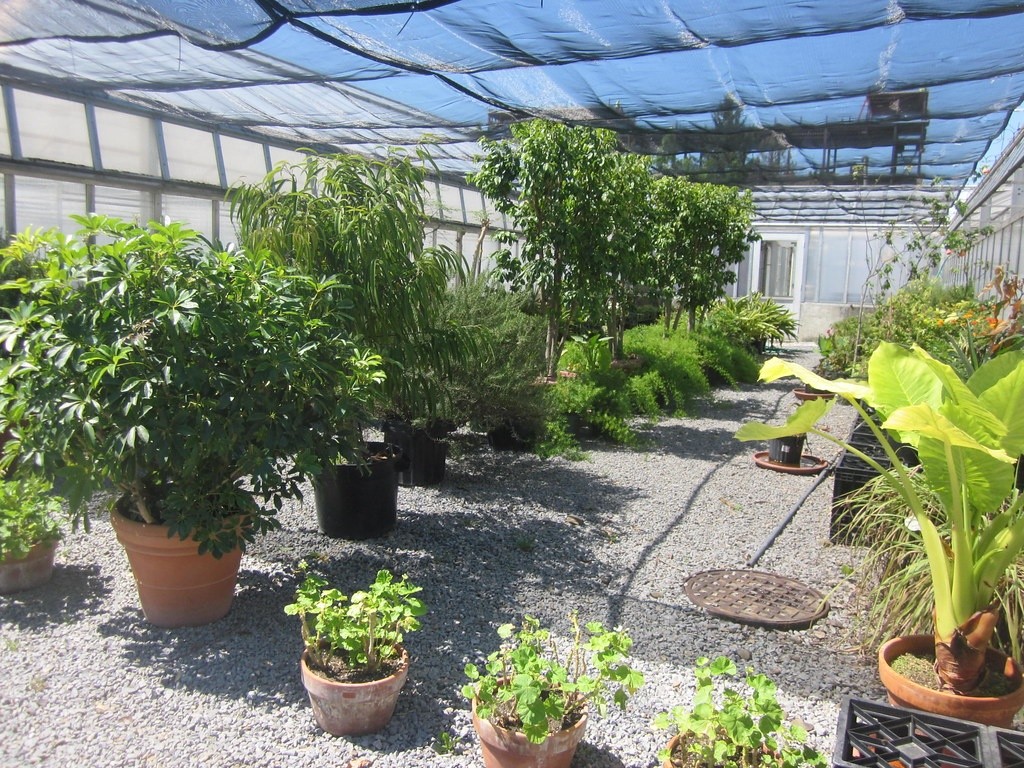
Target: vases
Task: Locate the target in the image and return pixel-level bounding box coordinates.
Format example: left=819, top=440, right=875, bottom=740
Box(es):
left=768, top=433, right=807, bottom=466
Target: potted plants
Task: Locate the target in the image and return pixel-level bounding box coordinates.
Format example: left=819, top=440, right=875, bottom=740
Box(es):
left=1, top=472, right=65, bottom=590
left=465, top=113, right=765, bottom=445
left=793, top=382, right=834, bottom=409
left=461, top=608, right=646, bottom=768
left=447, top=269, right=546, bottom=452
left=733, top=339, right=1024, bottom=733
left=383, top=247, right=495, bottom=489
left=221, top=138, right=432, bottom=541
left=654, top=654, right=826, bottom=768
left=282, top=555, right=428, bottom=735
left=1, top=209, right=389, bottom=628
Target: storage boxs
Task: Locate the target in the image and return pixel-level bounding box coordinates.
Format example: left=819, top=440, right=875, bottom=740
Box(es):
left=830, top=694, right=1024, bottom=768
